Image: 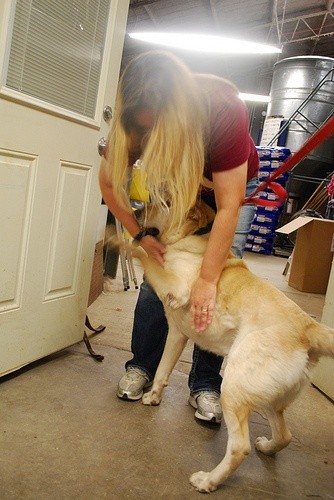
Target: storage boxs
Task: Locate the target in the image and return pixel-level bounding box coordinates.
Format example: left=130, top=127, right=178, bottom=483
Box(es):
left=259, top=115, right=288, bottom=147
left=275, top=216, right=334, bottom=295
left=243, top=146, right=292, bottom=255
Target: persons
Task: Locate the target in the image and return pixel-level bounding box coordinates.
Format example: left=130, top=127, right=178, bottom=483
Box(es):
left=96, top=52, right=259, bottom=425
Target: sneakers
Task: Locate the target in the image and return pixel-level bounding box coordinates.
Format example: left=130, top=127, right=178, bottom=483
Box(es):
left=188, top=386, right=223, bottom=423
left=117, top=365, right=153, bottom=401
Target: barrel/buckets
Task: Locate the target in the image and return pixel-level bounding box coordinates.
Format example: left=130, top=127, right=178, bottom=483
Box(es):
left=229, top=205, right=257, bottom=259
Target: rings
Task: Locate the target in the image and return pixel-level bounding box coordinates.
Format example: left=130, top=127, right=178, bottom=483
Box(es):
left=202, top=308, right=207, bottom=312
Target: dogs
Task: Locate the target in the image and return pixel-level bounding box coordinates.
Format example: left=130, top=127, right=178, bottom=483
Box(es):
left=105, top=184, right=333, bottom=493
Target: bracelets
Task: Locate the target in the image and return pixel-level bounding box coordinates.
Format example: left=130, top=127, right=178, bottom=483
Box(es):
left=133, top=227, right=160, bottom=245
